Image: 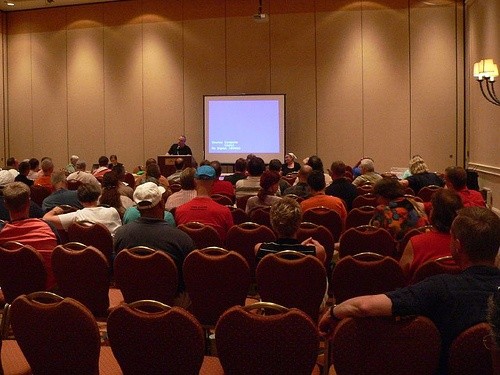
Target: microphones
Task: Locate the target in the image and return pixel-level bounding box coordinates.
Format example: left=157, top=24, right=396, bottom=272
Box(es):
left=176, top=146, right=180, bottom=150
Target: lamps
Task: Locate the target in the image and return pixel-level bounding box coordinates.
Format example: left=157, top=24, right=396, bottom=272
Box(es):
left=473, top=58, right=500, bottom=107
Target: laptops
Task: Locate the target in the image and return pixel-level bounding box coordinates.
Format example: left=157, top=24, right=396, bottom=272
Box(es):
left=86, top=163, right=99, bottom=173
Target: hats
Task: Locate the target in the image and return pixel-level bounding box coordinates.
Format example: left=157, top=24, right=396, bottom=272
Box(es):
left=0, top=170, right=14, bottom=187
left=9, top=168, right=20, bottom=178
left=288, top=152, right=297, bottom=160
left=133, top=181, right=166, bottom=209
left=196, top=165, right=215, bottom=180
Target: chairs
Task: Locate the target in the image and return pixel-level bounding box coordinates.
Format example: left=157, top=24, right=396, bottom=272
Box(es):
left=0, top=185, right=500, bottom=375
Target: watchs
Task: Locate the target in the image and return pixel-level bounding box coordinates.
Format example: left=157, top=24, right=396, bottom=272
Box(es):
left=330, top=304, right=342, bottom=322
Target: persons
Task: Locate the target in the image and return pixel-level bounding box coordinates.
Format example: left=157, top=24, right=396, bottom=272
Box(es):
left=224, top=153, right=358, bottom=237
left=318, top=207, right=500, bottom=375
left=107, top=181, right=196, bottom=310
left=0, top=155, right=235, bottom=244
left=165, top=135, right=192, bottom=155
left=255, top=196, right=327, bottom=268
left=352, top=155, right=487, bottom=277
